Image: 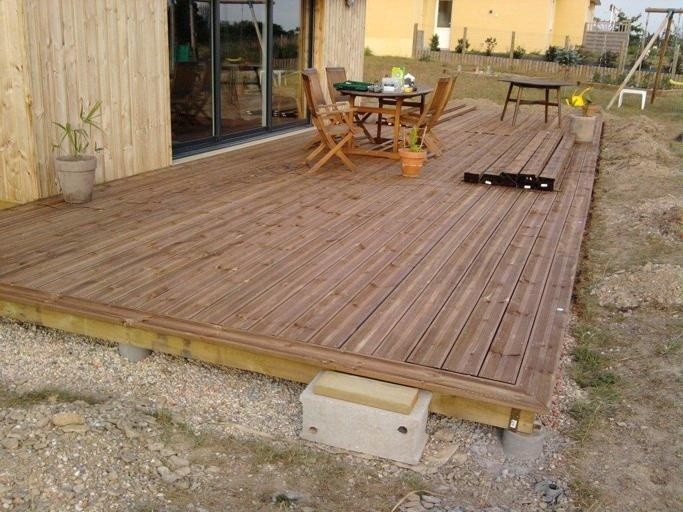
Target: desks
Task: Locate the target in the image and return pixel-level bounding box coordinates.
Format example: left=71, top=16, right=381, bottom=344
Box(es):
left=218, top=64, right=261, bottom=102
left=496, top=78, right=575, bottom=130
left=335, top=80, right=433, bottom=160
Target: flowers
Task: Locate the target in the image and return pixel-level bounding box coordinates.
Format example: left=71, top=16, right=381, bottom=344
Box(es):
left=562, top=86, right=598, bottom=117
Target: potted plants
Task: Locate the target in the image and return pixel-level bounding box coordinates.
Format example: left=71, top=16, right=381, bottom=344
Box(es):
left=44, top=94, right=110, bottom=205
left=398, top=123, right=426, bottom=179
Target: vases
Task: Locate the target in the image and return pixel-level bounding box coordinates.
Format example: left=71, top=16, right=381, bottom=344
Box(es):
left=566, top=113, right=595, bottom=143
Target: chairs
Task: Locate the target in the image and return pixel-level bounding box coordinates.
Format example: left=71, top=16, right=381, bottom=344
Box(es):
left=301, top=68, right=360, bottom=148
left=170, top=59, right=199, bottom=132
left=299, top=73, right=363, bottom=175
left=270, top=72, right=301, bottom=119
left=390, top=74, right=459, bottom=160
left=188, top=62, right=210, bottom=126
left=325, top=67, right=375, bottom=144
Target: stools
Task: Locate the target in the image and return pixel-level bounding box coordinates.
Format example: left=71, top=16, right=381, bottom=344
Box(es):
left=618, top=86, right=648, bottom=111
